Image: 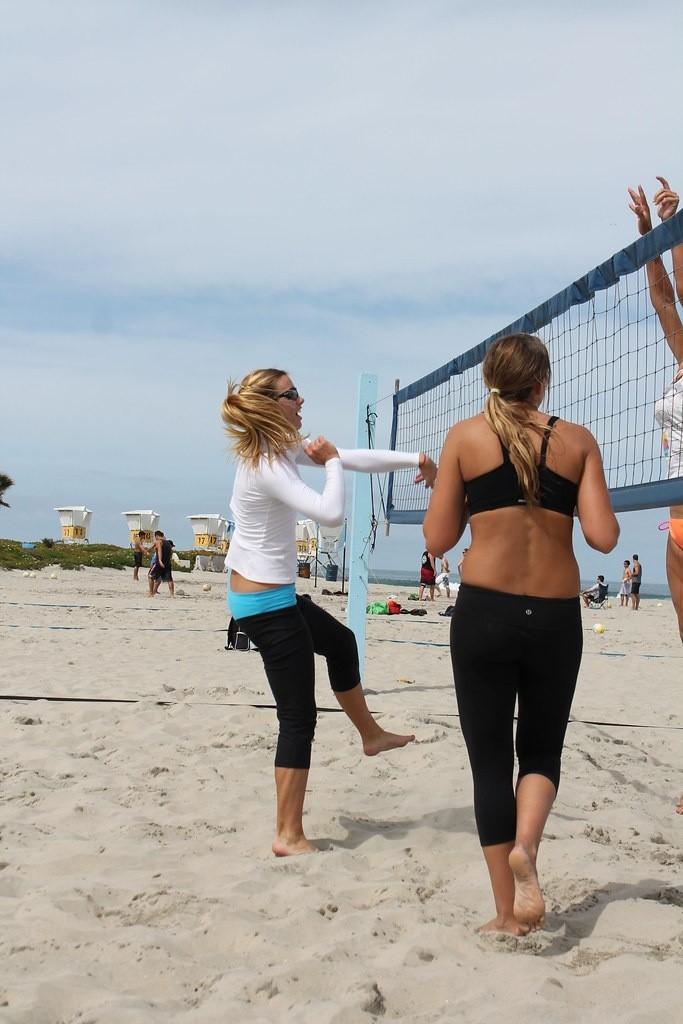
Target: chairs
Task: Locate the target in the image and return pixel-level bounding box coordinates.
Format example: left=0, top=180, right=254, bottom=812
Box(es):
left=581, top=583, right=610, bottom=610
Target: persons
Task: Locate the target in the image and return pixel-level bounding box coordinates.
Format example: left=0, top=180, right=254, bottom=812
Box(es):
left=627, top=178, right=683, bottom=815
left=619, top=560, right=631, bottom=606
left=582, top=575, right=604, bottom=608
left=134, top=531, right=149, bottom=580
left=423, top=334, right=620, bottom=934
left=145, top=530, right=176, bottom=597
left=435, top=554, right=450, bottom=598
left=629, top=554, right=642, bottom=610
left=458, top=548, right=468, bottom=576
left=419, top=551, right=437, bottom=601
left=220, top=369, right=438, bottom=855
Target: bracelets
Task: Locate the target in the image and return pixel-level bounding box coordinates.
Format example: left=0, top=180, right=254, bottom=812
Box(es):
left=420, top=454, right=426, bottom=467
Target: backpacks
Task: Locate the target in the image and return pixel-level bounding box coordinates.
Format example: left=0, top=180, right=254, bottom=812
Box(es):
left=225, top=616, right=250, bottom=651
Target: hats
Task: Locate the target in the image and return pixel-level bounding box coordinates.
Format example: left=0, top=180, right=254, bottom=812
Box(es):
left=139, top=531, right=146, bottom=535
left=168, top=540, right=175, bottom=547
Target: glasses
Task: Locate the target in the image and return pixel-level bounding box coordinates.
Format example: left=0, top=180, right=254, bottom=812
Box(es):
left=461, top=552, right=465, bottom=553
left=623, top=563, right=627, bottom=565
left=272, top=387, right=298, bottom=401
left=597, top=578, right=599, bottom=580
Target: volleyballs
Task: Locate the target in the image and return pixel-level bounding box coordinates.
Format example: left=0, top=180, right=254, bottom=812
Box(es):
left=605, top=600, right=613, bottom=609
left=50, top=573, right=58, bottom=580
left=202, top=583, right=212, bottom=592
left=591, top=622, right=606, bottom=635
left=22, top=570, right=37, bottom=578
left=656, top=602, right=663, bottom=608
